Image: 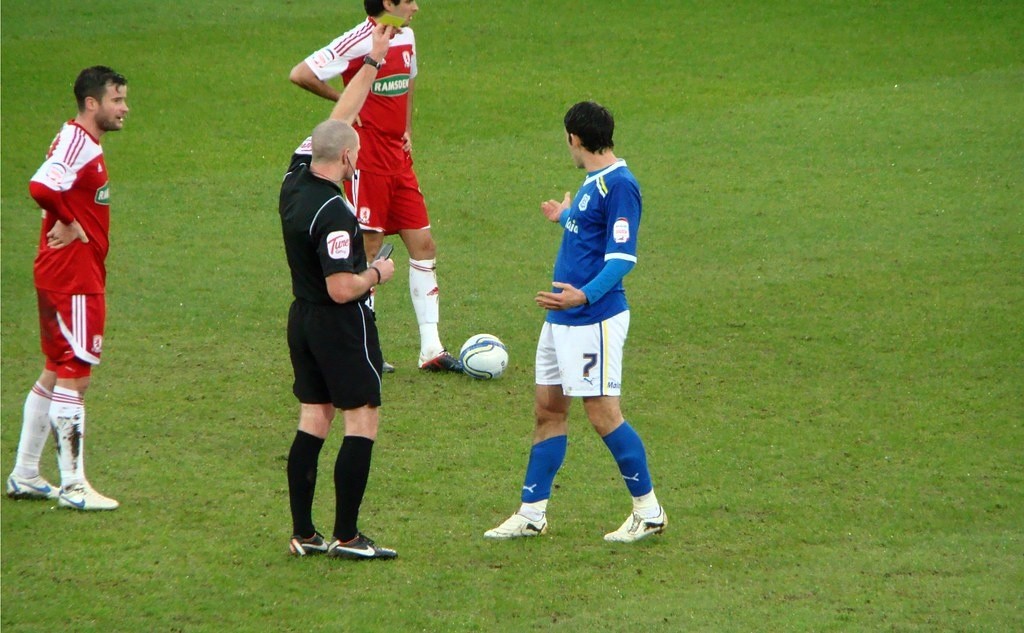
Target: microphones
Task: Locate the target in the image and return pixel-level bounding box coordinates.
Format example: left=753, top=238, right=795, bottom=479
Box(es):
left=347, top=157, right=357, bottom=179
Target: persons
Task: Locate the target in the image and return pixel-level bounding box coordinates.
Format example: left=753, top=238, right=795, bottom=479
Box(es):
left=5, top=66, right=132, bottom=511
left=278, top=22, right=407, bottom=558
left=289, top=1, right=465, bottom=374
left=481, top=98, right=668, bottom=544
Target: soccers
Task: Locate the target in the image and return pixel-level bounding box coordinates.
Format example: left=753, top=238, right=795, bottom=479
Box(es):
left=459, top=333, right=510, bottom=381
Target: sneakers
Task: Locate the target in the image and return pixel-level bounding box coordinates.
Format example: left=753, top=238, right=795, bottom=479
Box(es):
left=382, top=360, right=394, bottom=373
left=483, top=511, right=549, bottom=538
left=288, top=534, right=331, bottom=555
left=601, top=505, right=668, bottom=543
left=58, top=487, right=119, bottom=511
left=418, top=351, right=464, bottom=373
left=328, top=532, right=399, bottom=560
left=6, top=473, right=59, bottom=498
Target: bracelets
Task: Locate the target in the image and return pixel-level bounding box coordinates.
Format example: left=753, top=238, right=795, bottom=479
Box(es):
left=363, top=55, right=381, bottom=70
left=369, top=267, right=382, bottom=286
